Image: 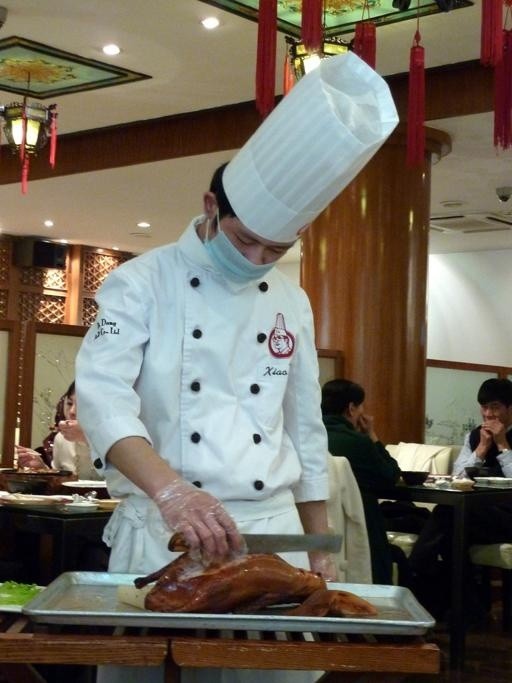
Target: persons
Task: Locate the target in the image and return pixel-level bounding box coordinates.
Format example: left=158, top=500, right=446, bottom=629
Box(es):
left=408, top=379, right=512, bottom=570
left=321, top=378, right=420, bottom=606
left=17, top=381, right=98, bottom=482
left=74, top=51, right=399, bottom=682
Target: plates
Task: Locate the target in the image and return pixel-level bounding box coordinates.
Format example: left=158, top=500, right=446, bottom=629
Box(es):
left=1, top=584, right=48, bottom=613
left=472, top=476, right=512, bottom=483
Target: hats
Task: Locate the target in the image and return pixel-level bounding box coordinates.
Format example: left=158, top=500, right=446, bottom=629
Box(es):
left=220, top=51, right=399, bottom=245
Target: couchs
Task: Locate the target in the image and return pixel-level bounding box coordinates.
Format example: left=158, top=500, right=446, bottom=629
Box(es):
left=327, top=451, right=373, bottom=584
left=387, top=443, right=511, bottom=631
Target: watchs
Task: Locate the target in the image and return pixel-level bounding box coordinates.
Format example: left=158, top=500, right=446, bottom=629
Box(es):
left=495, top=449, right=511, bottom=455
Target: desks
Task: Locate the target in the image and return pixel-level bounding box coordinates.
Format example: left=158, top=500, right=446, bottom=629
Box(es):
left=1, top=611, right=442, bottom=683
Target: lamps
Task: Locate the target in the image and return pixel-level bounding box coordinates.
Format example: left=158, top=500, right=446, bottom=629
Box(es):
left=0, top=71, right=58, bottom=194
left=285, top=1, right=355, bottom=86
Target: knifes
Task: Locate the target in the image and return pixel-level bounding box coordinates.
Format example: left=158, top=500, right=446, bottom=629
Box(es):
left=168, top=532, right=343, bottom=555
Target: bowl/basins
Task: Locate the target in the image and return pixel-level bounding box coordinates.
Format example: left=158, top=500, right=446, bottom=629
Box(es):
left=62, top=481, right=107, bottom=499
left=400, top=471, right=430, bottom=485
left=0, top=468, right=72, bottom=494
left=64, top=502, right=101, bottom=514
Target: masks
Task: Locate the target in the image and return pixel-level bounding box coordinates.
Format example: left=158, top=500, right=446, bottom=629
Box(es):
left=201, top=206, right=280, bottom=286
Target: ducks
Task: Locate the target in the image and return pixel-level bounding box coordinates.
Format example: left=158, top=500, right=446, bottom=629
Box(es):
left=134, top=533, right=380, bottom=618
